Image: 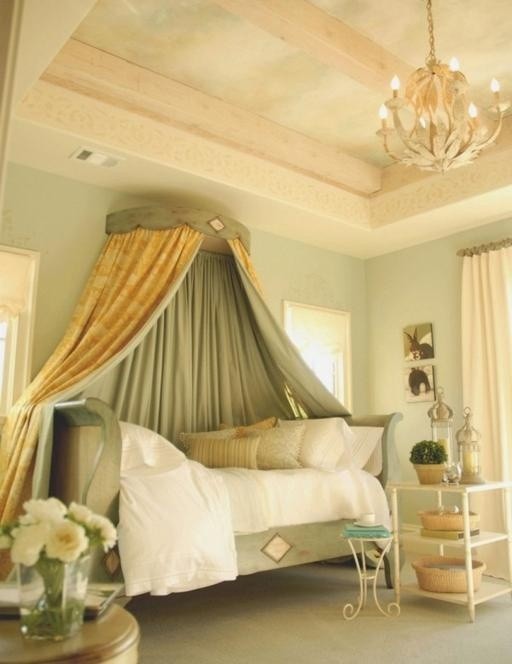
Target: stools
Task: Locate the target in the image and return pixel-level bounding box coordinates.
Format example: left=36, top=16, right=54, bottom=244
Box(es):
left=340, top=531, right=401, bottom=620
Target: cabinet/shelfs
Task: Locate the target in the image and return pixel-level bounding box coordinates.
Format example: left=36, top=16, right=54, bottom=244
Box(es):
left=389, top=480, right=512, bottom=622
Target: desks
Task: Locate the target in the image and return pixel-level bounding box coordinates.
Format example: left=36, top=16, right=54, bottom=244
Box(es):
left=0, top=602, right=140, bottom=663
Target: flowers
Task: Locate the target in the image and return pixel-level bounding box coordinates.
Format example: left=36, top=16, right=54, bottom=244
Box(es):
left=0, top=495, right=119, bottom=634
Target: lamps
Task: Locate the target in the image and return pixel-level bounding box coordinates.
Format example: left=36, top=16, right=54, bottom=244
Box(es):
left=373, top=0, right=511, bottom=173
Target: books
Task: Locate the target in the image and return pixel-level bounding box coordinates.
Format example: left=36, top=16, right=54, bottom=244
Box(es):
left=421, top=529, right=480, bottom=540
left=0, top=582, right=124, bottom=620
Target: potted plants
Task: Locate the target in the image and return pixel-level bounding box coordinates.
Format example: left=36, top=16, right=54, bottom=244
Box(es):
left=409, top=439, right=449, bottom=484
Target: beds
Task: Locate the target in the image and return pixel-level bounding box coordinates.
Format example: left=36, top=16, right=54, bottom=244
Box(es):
left=8, top=395, right=403, bottom=609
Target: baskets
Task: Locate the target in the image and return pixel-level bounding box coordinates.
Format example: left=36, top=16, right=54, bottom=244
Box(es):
left=415, top=463, right=445, bottom=484
left=412, top=557, right=486, bottom=593
left=418, top=510, right=478, bottom=530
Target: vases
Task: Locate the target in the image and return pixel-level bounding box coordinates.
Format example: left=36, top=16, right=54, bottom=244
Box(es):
left=16, top=552, right=93, bottom=641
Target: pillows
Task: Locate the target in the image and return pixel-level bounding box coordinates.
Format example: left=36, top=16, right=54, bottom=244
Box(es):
left=175, top=414, right=384, bottom=476
